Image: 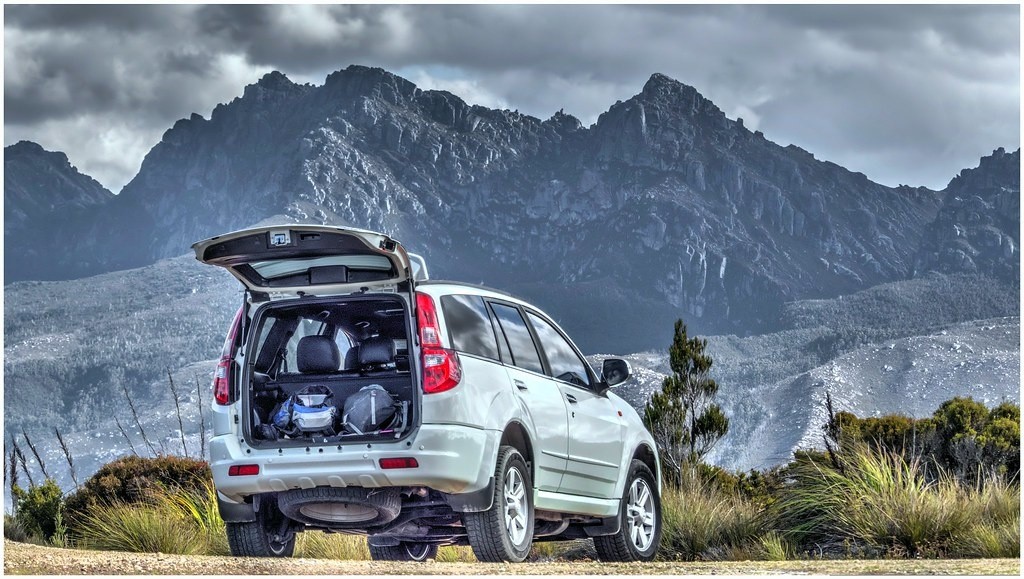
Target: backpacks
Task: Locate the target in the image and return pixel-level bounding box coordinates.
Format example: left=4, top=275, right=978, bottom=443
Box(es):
left=269, top=384, right=339, bottom=439
left=338, top=384, right=408, bottom=439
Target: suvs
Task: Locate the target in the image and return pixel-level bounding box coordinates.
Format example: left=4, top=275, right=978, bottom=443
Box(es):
left=188, top=224, right=664, bottom=562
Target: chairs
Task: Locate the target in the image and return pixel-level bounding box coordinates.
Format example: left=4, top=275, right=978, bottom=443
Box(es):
left=359, top=336, right=399, bottom=375
left=295, top=335, right=342, bottom=373
left=345, top=346, right=360, bottom=370
